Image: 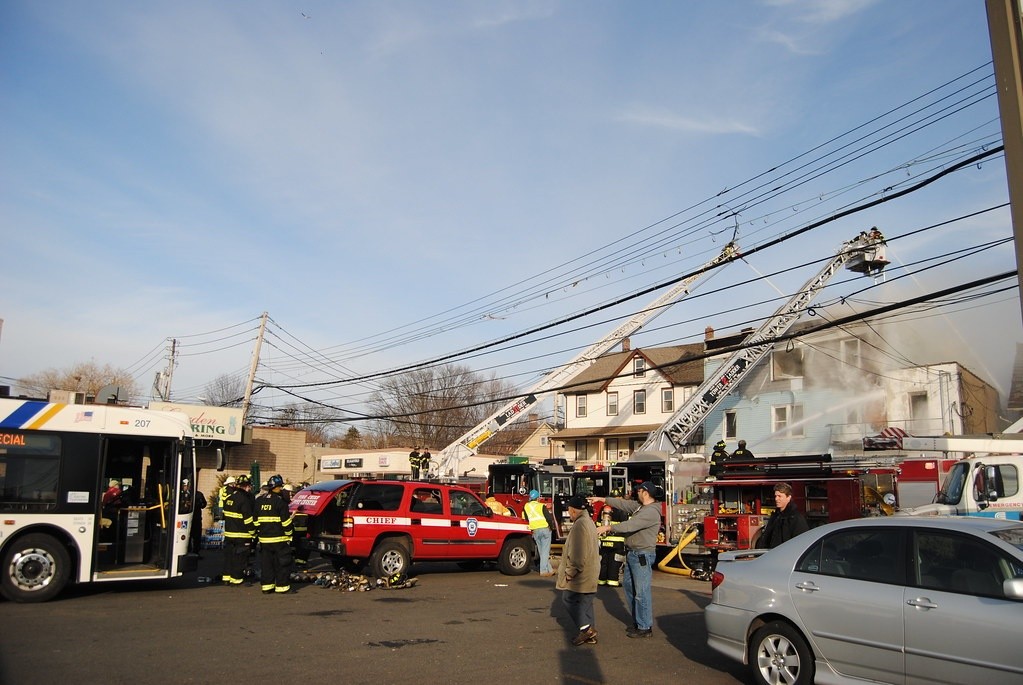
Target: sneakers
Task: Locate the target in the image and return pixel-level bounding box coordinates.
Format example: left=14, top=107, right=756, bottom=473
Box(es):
left=627, top=625, right=638, bottom=632
left=628, top=629, right=654, bottom=638
left=572, top=625, right=598, bottom=646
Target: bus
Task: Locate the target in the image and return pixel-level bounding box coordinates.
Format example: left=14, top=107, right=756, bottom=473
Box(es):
left=0, top=396, right=228, bottom=605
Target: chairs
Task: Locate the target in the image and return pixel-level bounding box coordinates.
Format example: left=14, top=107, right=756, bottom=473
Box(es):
left=412, top=497, right=425, bottom=512
left=918, top=544, right=1005, bottom=597
left=816, top=539, right=895, bottom=583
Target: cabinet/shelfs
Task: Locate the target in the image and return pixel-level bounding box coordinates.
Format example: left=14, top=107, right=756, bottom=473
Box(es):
left=201, top=521, right=226, bottom=549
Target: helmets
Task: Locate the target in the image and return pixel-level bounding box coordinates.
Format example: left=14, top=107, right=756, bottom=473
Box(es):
left=182, top=478, right=191, bottom=486
left=713, top=440, right=726, bottom=450
left=268, top=476, right=284, bottom=489
left=738, top=440, right=747, bottom=446
left=283, top=485, right=294, bottom=491
left=529, top=490, right=539, bottom=500
left=261, top=481, right=268, bottom=489
left=414, top=446, right=420, bottom=450
left=223, top=476, right=236, bottom=484
left=236, top=475, right=251, bottom=485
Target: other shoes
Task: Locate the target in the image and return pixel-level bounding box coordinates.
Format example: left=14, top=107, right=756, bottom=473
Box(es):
left=197, top=569, right=418, bottom=593
left=608, top=582, right=623, bottom=587
left=540, top=570, right=556, bottom=577
left=598, top=584, right=607, bottom=586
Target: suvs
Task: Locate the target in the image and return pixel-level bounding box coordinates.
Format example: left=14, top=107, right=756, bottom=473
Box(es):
left=287, top=476, right=537, bottom=587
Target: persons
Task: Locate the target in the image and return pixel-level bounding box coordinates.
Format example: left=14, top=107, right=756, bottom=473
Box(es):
left=409, top=445, right=431, bottom=481
left=181, top=478, right=207, bottom=558
left=850, top=226, right=884, bottom=243
left=101, top=478, right=124, bottom=538
left=220, top=475, right=256, bottom=587
left=216, top=475, right=291, bottom=552
left=484, top=493, right=511, bottom=516
left=709, top=439, right=756, bottom=510
left=555, top=496, right=600, bottom=645
left=725, top=242, right=734, bottom=248
left=524, top=489, right=556, bottom=576
left=253, top=476, right=299, bottom=595
left=586, top=482, right=664, bottom=638
left=754, top=481, right=809, bottom=558
left=598, top=487, right=629, bottom=586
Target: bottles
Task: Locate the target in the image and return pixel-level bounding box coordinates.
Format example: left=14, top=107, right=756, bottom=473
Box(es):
left=678, top=497, right=714, bottom=529
left=201, top=520, right=223, bottom=549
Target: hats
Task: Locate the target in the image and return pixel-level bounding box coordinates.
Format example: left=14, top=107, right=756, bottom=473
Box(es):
left=565, top=496, right=589, bottom=509
left=109, top=480, right=122, bottom=487
left=635, top=481, right=664, bottom=498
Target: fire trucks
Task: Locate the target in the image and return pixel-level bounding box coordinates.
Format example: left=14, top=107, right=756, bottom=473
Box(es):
left=414, top=226, right=984, bottom=580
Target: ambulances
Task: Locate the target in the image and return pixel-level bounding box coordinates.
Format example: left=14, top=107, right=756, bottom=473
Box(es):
left=703, top=514, right=1023, bottom=685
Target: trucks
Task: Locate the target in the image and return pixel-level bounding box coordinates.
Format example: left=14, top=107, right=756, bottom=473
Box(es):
left=894, top=437, right=1023, bottom=523
